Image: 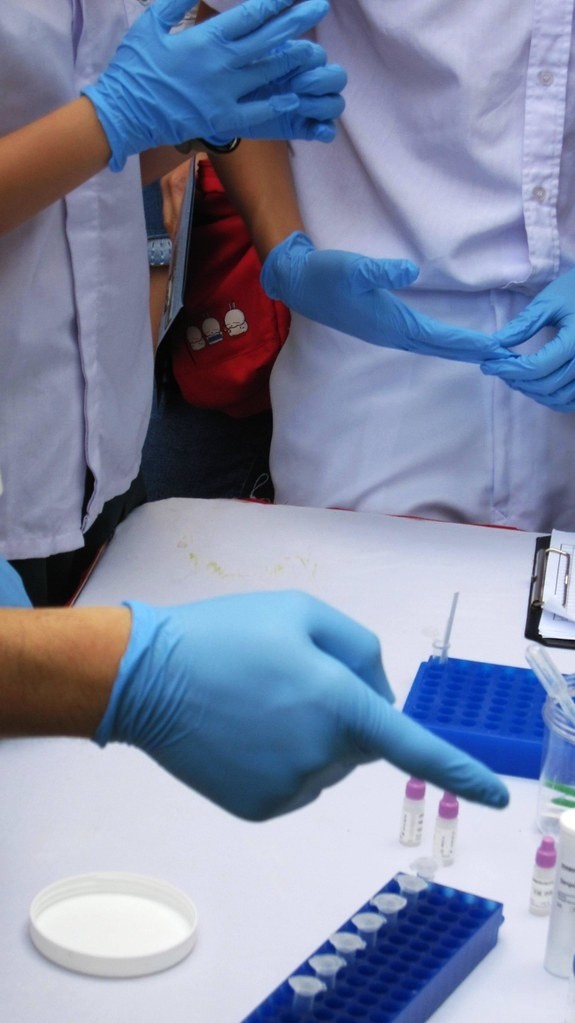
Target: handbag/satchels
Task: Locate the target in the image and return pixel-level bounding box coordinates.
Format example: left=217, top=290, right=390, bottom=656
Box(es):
left=175, top=171, right=284, bottom=421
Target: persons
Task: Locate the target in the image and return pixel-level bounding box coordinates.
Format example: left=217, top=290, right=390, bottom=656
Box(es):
left=0, top=0, right=349, bottom=605
left=0, top=594, right=512, bottom=822
left=204, top=1, right=575, bottom=533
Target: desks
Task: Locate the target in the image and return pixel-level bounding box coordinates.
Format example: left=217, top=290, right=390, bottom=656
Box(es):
left=0, top=496, right=575, bottom=1023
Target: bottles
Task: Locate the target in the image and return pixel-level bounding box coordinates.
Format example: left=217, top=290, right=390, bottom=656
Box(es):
left=532, top=673, right=575, bottom=841
left=544, top=808, right=575, bottom=981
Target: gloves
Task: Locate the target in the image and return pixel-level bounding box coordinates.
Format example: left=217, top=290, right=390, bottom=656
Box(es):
left=79, top=0, right=351, bottom=176
left=259, top=230, right=515, bottom=363
left=482, top=263, right=575, bottom=412
left=95, top=590, right=509, bottom=823
